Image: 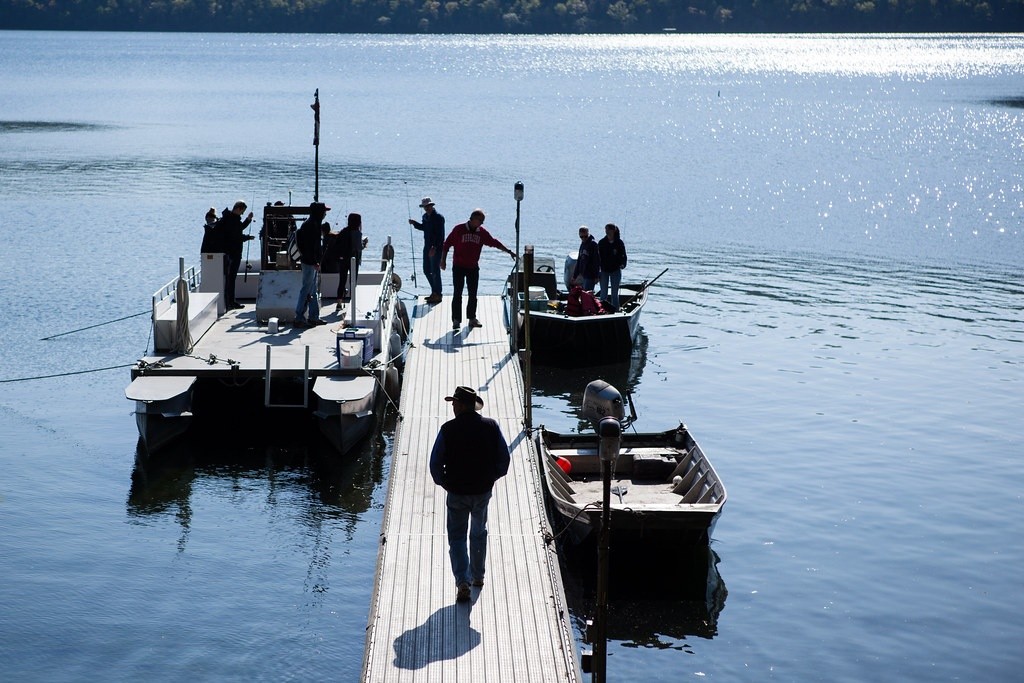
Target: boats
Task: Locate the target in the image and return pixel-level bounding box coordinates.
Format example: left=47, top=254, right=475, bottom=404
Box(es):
left=507, top=256, right=650, bottom=346
left=538, top=379, right=726, bottom=540
left=124, top=202, right=409, bottom=456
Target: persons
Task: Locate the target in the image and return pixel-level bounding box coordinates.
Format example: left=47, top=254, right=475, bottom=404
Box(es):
left=597, top=224, right=627, bottom=309
left=293, top=202, right=327, bottom=328
left=336, top=213, right=369, bottom=310
left=408, top=196, right=445, bottom=303
left=258, top=201, right=297, bottom=262
left=221, top=201, right=256, bottom=310
left=571, top=226, right=600, bottom=291
left=440, top=209, right=521, bottom=330
left=430, top=386, right=510, bottom=603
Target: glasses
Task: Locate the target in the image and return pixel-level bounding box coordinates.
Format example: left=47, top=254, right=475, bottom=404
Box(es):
left=580, top=236, right=588, bottom=238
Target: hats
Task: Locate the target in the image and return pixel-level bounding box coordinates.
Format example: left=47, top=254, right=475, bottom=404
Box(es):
left=305, top=202, right=331, bottom=214
left=445, top=387, right=483, bottom=410
left=419, top=197, right=435, bottom=207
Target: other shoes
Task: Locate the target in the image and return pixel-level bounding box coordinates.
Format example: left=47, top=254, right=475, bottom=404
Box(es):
left=457, top=583, right=471, bottom=603
left=226, top=302, right=245, bottom=309
left=472, top=578, right=483, bottom=586
left=469, top=319, right=482, bottom=328
left=424, top=294, right=442, bottom=303
left=307, top=318, right=328, bottom=325
left=293, top=320, right=315, bottom=328
left=453, top=323, right=460, bottom=329
